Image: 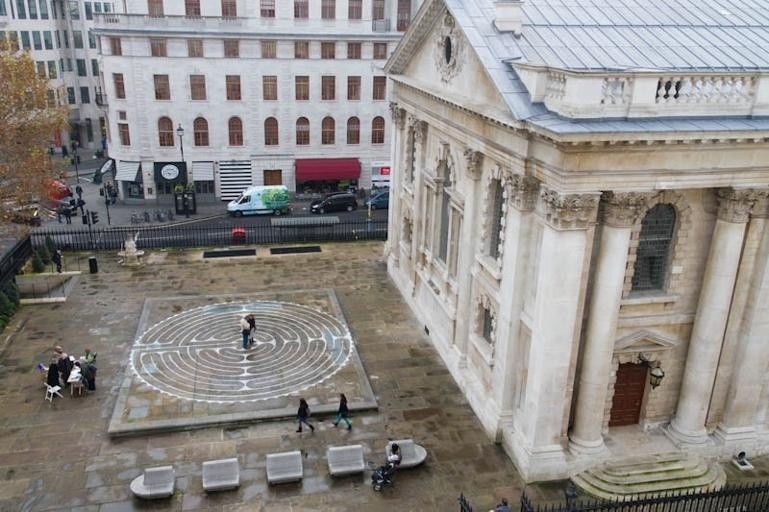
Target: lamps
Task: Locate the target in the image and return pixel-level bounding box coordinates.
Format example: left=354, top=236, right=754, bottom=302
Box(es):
left=650, top=361, right=665, bottom=390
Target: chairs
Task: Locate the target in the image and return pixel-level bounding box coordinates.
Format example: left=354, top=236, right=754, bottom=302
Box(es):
left=43, top=383, right=64, bottom=402
left=39, top=364, right=49, bottom=381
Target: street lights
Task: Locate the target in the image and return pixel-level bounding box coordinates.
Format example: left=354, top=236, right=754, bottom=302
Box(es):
left=639, top=352, right=664, bottom=389
left=176, top=122, right=190, bottom=217
left=72, top=141, right=80, bottom=184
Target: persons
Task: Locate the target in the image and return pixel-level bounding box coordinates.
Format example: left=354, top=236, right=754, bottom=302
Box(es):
left=49, top=140, right=118, bottom=226
left=248, top=313, right=257, bottom=345
left=387, top=442, right=404, bottom=471
left=51, top=248, right=65, bottom=273
left=331, top=393, right=352, bottom=431
left=37, top=342, right=99, bottom=400
left=295, top=398, right=315, bottom=433
left=240, top=314, right=251, bottom=349
left=493, top=496, right=513, bottom=512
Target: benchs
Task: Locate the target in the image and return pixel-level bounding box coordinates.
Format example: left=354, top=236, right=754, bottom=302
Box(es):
left=386, top=439, right=428, bottom=469
left=264, top=451, right=303, bottom=486
left=130, top=466, right=178, bottom=499
left=327, top=446, right=366, bottom=477
left=201, top=458, right=240, bottom=493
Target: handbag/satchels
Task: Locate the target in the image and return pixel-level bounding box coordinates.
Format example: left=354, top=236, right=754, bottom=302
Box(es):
left=243, top=329, right=250, bottom=335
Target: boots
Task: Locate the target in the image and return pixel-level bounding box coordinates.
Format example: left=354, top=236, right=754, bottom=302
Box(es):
left=297, top=427, right=302, bottom=432
left=310, top=425, right=314, bottom=432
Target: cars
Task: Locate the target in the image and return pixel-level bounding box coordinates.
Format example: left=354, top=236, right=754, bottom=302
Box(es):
left=310, top=192, right=358, bottom=214
left=364, top=188, right=389, bottom=210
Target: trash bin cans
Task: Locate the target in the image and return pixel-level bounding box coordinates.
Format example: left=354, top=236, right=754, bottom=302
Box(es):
left=100, top=188, right=104, bottom=196
left=89, top=256, right=97, bottom=274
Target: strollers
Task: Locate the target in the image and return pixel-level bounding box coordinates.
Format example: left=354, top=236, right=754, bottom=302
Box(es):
left=372, top=462, right=395, bottom=492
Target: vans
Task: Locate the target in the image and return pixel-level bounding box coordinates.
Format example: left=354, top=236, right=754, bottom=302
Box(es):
left=227, top=184, right=288, bottom=217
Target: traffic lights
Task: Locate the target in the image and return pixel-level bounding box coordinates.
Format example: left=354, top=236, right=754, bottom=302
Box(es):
left=90, top=210, right=99, bottom=225
left=82, top=215, right=88, bottom=224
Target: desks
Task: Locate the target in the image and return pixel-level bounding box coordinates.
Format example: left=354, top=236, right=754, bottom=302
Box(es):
left=67, top=366, right=83, bottom=396
